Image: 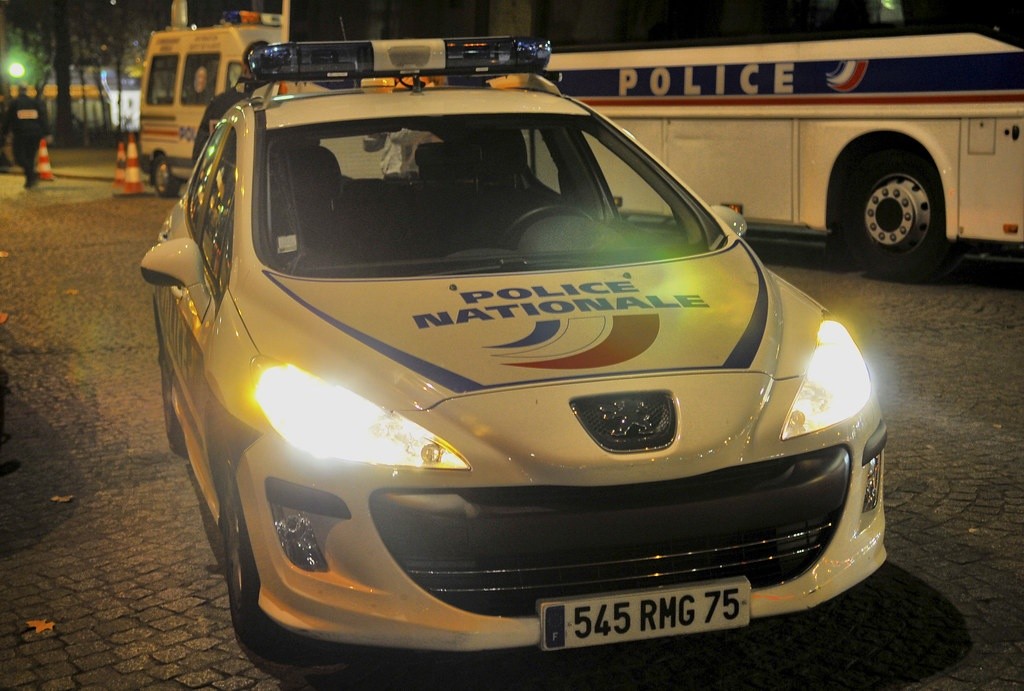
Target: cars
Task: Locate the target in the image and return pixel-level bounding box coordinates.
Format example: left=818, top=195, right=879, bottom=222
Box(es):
left=144, top=33, right=894, bottom=653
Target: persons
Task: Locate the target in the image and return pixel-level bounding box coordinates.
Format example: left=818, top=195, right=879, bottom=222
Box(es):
left=190, top=40, right=269, bottom=167
left=0, top=85, right=47, bottom=187
left=365, top=76, right=439, bottom=178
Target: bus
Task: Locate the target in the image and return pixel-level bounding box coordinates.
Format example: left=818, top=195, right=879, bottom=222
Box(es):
left=509, top=19, right=1024, bottom=278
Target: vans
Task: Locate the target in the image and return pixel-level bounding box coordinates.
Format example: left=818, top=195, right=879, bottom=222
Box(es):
left=139, top=11, right=365, bottom=198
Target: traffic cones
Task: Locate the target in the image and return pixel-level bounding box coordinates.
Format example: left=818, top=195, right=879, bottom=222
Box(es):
left=35, top=139, right=68, bottom=184
left=124, top=133, right=152, bottom=197
left=112, top=141, right=132, bottom=197
